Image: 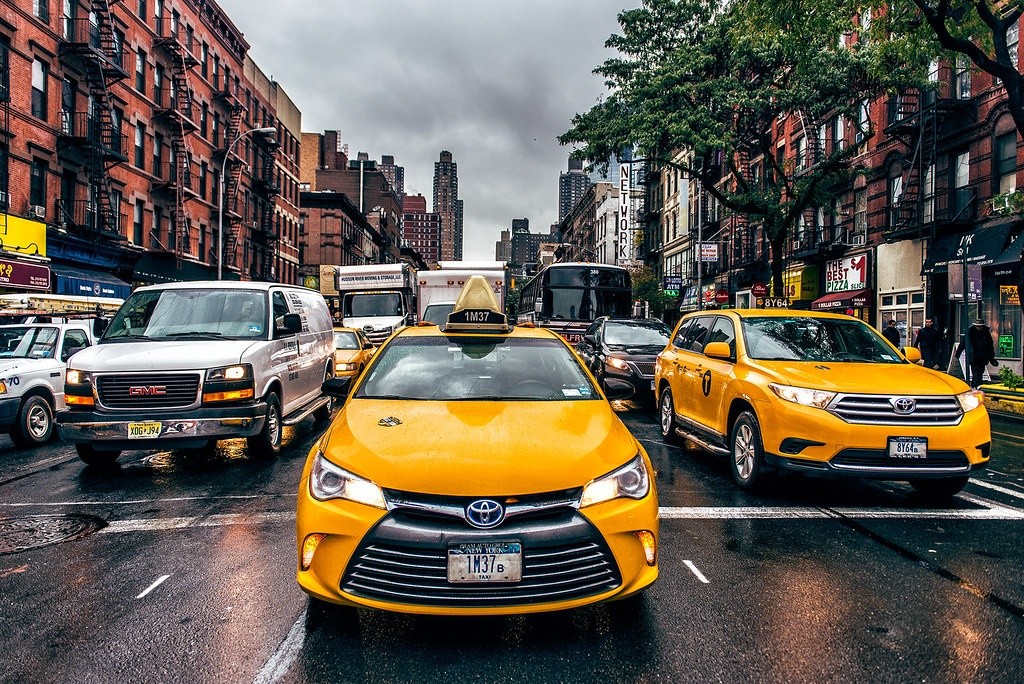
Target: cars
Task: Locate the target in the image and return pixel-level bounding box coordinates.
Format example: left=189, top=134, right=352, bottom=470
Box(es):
left=0, top=324, right=99, bottom=448
left=652, top=308, right=994, bottom=495
left=296, top=322, right=659, bottom=616
left=326, top=327, right=378, bottom=395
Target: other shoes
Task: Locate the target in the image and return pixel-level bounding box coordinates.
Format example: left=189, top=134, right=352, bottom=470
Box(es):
left=977, top=386, right=981, bottom=390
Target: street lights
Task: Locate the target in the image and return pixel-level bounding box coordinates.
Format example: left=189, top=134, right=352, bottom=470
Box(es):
left=217, top=126, right=277, bottom=279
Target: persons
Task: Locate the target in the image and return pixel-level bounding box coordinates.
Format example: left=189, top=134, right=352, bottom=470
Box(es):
left=956, top=319, right=999, bottom=389
left=913, top=317, right=943, bottom=371
left=883, top=320, right=900, bottom=350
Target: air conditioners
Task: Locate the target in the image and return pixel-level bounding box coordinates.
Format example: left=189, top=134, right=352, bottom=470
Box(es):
left=254, top=123, right=261, bottom=129
left=794, top=241, right=800, bottom=249
left=245, top=166, right=252, bottom=172
left=0, top=192, right=12, bottom=209
left=30, top=205, right=45, bottom=219
left=853, top=235, right=864, bottom=247
left=291, top=154, right=295, bottom=161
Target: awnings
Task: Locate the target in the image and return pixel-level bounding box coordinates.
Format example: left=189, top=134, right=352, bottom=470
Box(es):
left=812, top=288, right=872, bottom=310
left=918, top=219, right=1024, bottom=277
left=0, top=255, right=132, bottom=299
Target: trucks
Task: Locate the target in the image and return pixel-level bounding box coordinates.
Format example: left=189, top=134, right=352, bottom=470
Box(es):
left=333, top=263, right=414, bottom=352
left=416, top=270, right=507, bottom=325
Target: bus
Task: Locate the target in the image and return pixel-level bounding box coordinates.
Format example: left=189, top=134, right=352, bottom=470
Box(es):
left=519, top=263, right=636, bottom=345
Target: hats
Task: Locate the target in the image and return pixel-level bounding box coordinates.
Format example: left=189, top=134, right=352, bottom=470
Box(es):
left=972, top=319, right=986, bottom=326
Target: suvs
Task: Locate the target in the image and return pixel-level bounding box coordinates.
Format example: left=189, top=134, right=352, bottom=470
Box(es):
left=583, top=315, right=671, bottom=405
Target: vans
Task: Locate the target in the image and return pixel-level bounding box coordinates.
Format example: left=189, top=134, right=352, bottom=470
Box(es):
left=54, top=281, right=336, bottom=457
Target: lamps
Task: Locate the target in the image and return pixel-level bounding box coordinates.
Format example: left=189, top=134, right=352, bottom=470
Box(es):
left=0, top=238, right=43, bottom=258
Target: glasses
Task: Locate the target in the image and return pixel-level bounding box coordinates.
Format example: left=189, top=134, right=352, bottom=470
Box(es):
left=892, top=324, right=895, bottom=325
